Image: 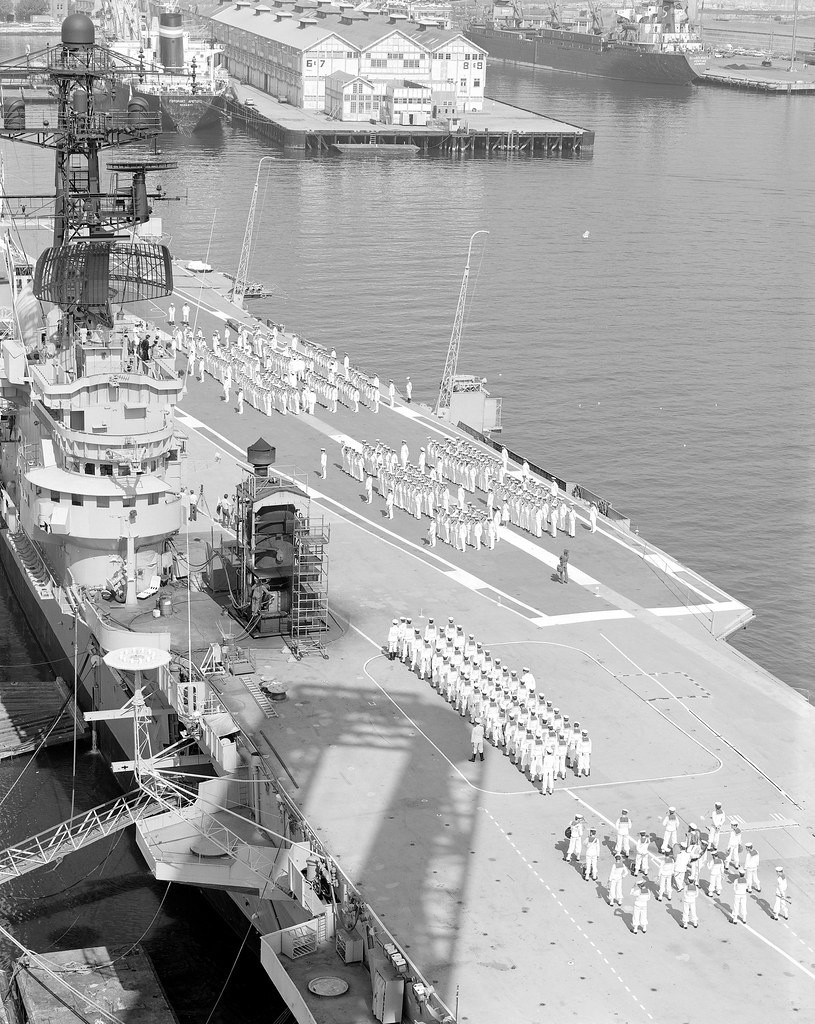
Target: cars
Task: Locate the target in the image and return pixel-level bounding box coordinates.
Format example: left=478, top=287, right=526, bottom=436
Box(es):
left=707, top=44, right=773, bottom=57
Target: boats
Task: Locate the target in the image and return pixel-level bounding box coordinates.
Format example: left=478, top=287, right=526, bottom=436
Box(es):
left=183, top=261, right=213, bottom=273
left=462, top=0, right=711, bottom=86
left=245, top=98, right=255, bottom=105
left=277, top=96, right=288, bottom=103
left=331, top=141, right=422, bottom=155
left=1, top=45, right=230, bottom=135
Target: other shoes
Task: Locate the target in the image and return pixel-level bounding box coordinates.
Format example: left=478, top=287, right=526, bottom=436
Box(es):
left=562, top=847, right=788, bottom=934
left=388, top=652, right=588, bottom=795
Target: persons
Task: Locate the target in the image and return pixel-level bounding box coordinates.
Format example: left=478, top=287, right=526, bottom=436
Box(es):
left=152, top=340, right=163, bottom=357
left=319, top=436, right=598, bottom=552
left=141, top=335, right=151, bottom=362
left=168, top=301, right=413, bottom=417
left=189, top=490, right=198, bottom=521
left=220, top=494, right=231, bottom=527
left=559, top=549, right=570, bottom=584
left=388, top=616, right=592, bottom=795
left=563, top=801, right=788, bottom=934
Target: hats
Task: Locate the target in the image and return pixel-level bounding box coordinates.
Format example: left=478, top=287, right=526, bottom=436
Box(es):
left=615, top=854, right=621, bottom=858
left=669, top=807, right=676, bottom=812
left=392, top=616, right=588, bottom=753
left=622, top=809, right=628, bottom=813
left=170, top=301, right=411, bottom=392
left=739, top=869, right=746, bottom=874
left=701, top=840, right=708, bottom=846
left=320, top=436, right=596, bottom=523
left=576, top=814, right=583, bottom=818
left=135, top=323, right=140, bottom=327
left=665, top=848, right=671, bottom=852
left=590, top=829, right=597, bottom=831
left=745, top=843, right=752, bottom=846
left=715, top=802, right=721, bottom=805
left=636, top=880, right=643, bottom=884
left=776, top=866, right=784, bottom=871
left=730, top=820, right=738, bottom=824
left=688, top=875, right=695, bottom=880
left=680, top=841, right=686, bottom=847
left=640, top=830, right=646, bottom=834
left=711, top=851, right=718, bottom=855
left=689, top=823, right=697, bottom=829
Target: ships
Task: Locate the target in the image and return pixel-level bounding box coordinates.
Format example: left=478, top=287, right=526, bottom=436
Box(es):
left=1, top=9, right=815, bottom=1024
